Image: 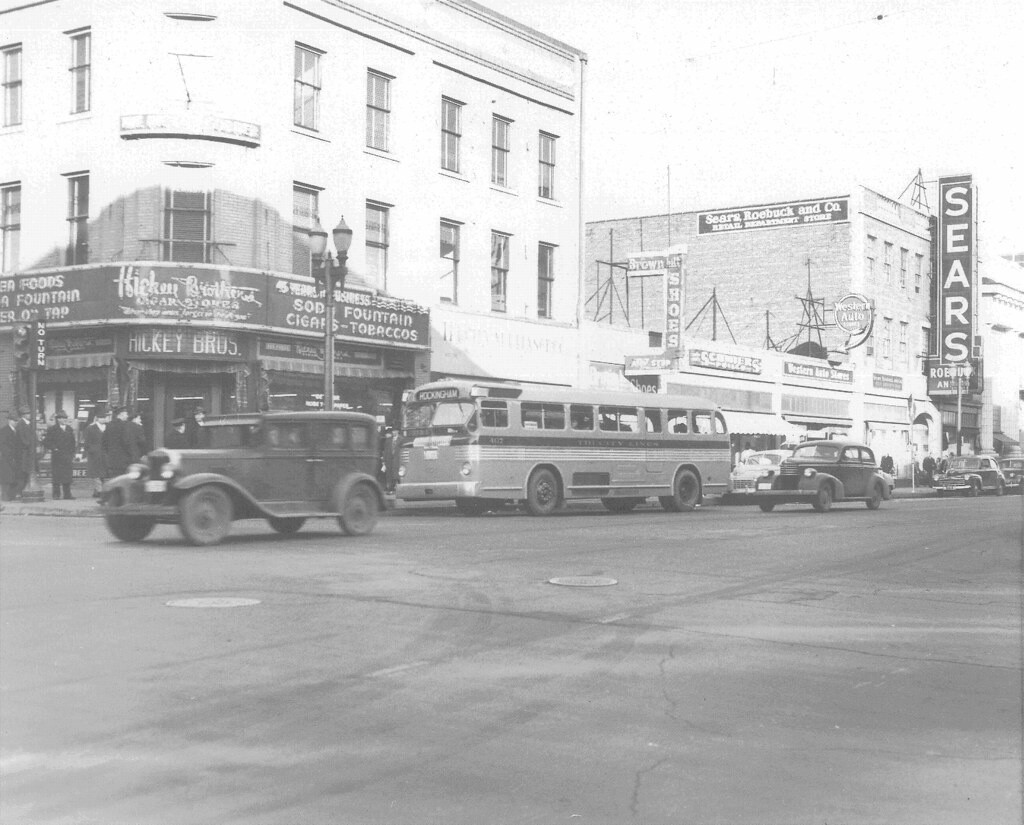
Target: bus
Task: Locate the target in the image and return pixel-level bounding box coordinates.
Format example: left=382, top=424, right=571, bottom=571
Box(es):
left=394, top=381, right=733, bottom=518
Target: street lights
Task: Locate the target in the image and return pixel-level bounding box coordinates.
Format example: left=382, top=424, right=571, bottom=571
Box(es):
left=308, top=214, right=353, bottom=414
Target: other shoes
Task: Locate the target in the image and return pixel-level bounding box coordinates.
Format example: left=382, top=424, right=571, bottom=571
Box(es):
left=387, top=490, right=394, bottom=495
left=53, top=495, right=60, bottom=500
left=63, top=495, right=76, bottom=499
left=92, top=490, right=100, bottom=498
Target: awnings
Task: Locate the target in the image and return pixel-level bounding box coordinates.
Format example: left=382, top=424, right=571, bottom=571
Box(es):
left=715, top=411, right=807, bottom=436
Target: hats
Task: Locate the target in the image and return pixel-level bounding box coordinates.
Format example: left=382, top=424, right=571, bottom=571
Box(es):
left=55, top=410, right=68, bottom=418
left=391, top=426, right=399, bottom=430
left=171, top=417, right=189, bottom=425
left=192, top=406, right=206, bottom=415
left=96, top=411, right=106, bottom=418
left=113, top=406, right=130, bottom=417
left=950, top=452, right=954, bottom=456
left=5, top=410, right=20, bottom=421
left=19, top=404, right=31, bottom=414
left=130, top=409, right=143, bottom=419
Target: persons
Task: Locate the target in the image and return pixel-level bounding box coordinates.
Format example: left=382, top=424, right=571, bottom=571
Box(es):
left=184, top=406, right=212, bottom=449
left=382, top=425, right=403, bottom=494
left=165, top=417, right=190, bottom=449
left=84, top=408, right=111, bottom=498
left=43, top=410, right=76, bottom=500
left=103, top=406, right=139, bottom=479
left=923, top=452, right=954, bottom=487
left=0, top=410, right=21, bottom=501
left=127, top=410, right=147, bottom=460
left=880, top=453, right=893, bottom=474
left=15, top=405, right=38, bottom=500
left=914, top=454, right=921, bottom=487
left=741, top=441, right=756, bottom=463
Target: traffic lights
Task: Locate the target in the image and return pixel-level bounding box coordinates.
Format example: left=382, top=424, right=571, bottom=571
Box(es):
left=13, top=320, right=32, bottom=373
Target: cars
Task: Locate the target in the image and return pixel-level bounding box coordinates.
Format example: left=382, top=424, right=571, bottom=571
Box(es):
left=752, top=440, right=889, bottom=513
left=876, top=470, right=895, bottom=501
left=91, top=411, right=394, bottom=546
left=931, top=455, right=1006, bottom=497
left=726, top=448, right=815, bottom=494
left=998, top=458, right=1024, bottom=495
